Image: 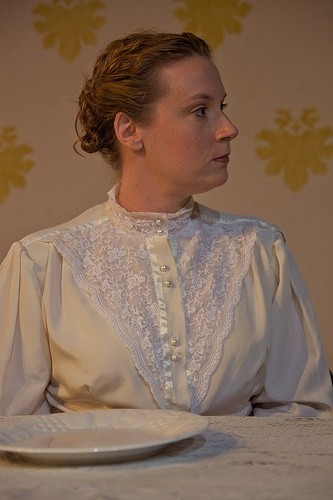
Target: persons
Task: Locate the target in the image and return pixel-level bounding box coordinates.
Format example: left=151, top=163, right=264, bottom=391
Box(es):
left=0, top=30, right=333, bottom=417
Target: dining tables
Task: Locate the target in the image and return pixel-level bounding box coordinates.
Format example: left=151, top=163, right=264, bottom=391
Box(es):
left=0, top=415, right=333, bottom=500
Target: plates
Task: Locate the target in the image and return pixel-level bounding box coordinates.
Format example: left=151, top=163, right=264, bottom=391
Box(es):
left=0, top=410, right=209, bottom=466
